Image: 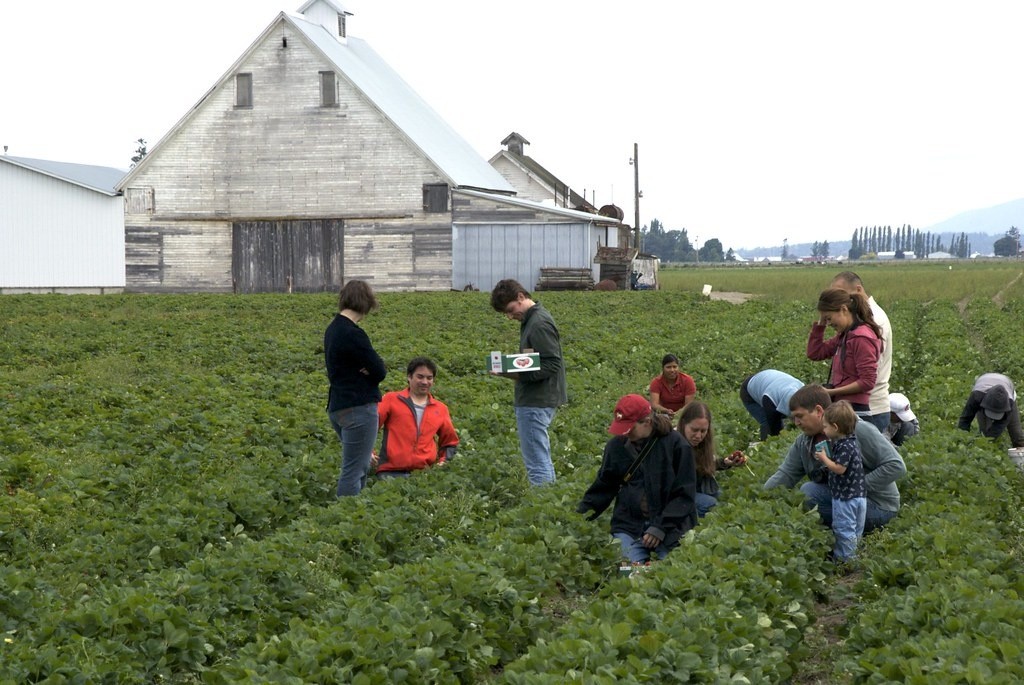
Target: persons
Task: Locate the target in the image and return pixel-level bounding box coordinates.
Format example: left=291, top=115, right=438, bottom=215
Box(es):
left=879, top=391, right=921, bottom=450
left=318, top=278, right=387, bottom=498
left=957, top=371, right=1023, bottom=453
left=759, top=384, right=908, bottom=536
left=827, top=272, right=892, bottom=435
left=806, top=287, right=885, bottom=433
left=668, top=398, right=748, bottom=526
left=740, top=369, right=805, bottom=440
left=578, top=393, right=699, bottom=569
left=646, top=354, right=699, bottom=415
left=366, top=357, right=459, bottom=483
left=810, top=399, right=870, bottom=565
left=488, top=279, right=570, bottom=490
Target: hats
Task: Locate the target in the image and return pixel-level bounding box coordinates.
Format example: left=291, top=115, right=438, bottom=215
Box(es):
left=889, top=393, right=916, bottom=423
left=984, top=409, right=1005, bottom=420
left=610, top=394, right=651, bottom=436
